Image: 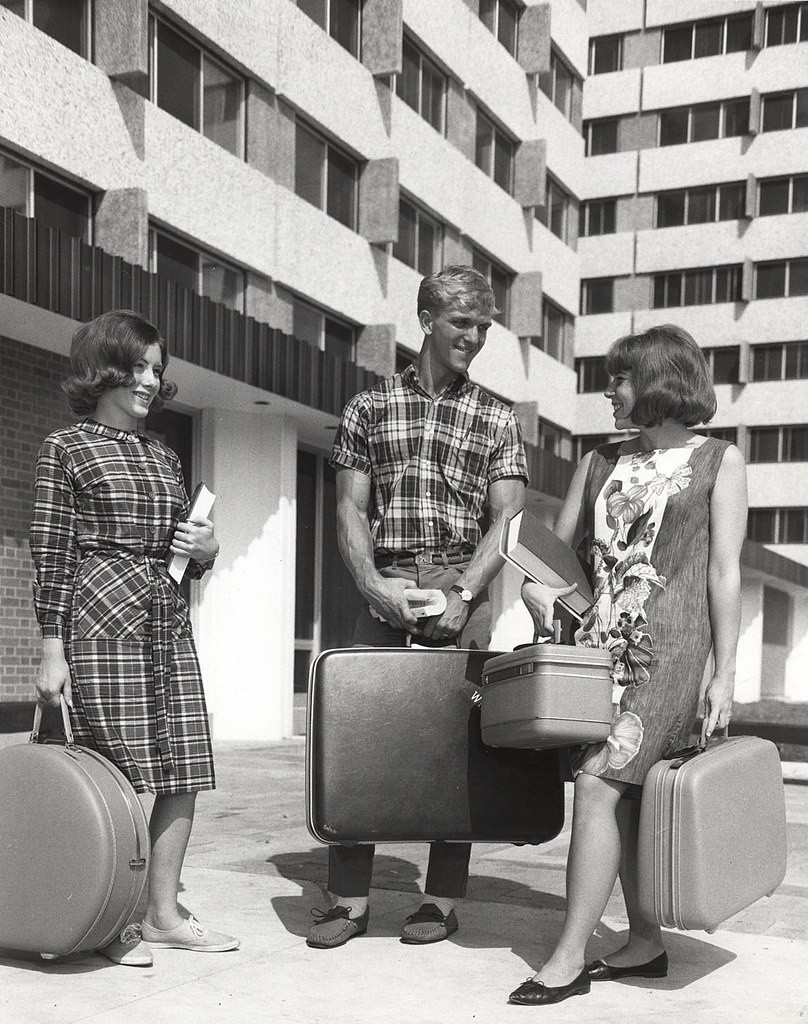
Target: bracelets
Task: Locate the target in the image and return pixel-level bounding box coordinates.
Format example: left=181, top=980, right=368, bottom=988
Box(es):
left=198, top=544, right=220, bottom=562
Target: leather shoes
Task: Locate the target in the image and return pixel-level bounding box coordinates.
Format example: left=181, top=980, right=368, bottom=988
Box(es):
left=587, top=950, right=669, bottom=981
left=509, top=966, right=591, bottom=1006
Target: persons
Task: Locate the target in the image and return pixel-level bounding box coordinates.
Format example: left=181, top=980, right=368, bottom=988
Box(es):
left=24, top=309, right=240, bottom=966
left=503, top=323, right=750, bottom=1006
left=305, top=259, right=529, bottom=948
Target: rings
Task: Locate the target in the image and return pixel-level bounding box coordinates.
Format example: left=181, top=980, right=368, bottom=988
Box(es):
left=47, top=699, right=52, bottom=702
left=443, top=633, right=448, bottom=637
left=726, top=715, right=733, bottom=719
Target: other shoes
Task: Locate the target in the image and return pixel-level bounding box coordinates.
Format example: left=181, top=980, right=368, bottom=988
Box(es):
left=305, top=904, right=370, bottom=949
left=400, top=903, right=458, bottom=944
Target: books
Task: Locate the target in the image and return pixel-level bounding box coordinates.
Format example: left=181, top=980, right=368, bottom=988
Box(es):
left=163, top=480, right=217, bottom=586
left=495, top=507, right=594, bottom=623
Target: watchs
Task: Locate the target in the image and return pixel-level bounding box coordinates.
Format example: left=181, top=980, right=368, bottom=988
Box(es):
left=450, top=584, right=476, bottom=605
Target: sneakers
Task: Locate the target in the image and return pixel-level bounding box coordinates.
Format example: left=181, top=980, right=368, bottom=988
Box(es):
left=98, top=923, right=154, bottom=965
left=141, top=915, right=239, bottom=952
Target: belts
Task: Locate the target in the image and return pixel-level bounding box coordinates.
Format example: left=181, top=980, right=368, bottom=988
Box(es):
left=391, top=554, right=475, bottom=566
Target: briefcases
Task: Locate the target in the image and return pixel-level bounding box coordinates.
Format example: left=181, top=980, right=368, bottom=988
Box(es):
left=0, top=695, right=150, bottom=960
left=306, top=627, right=566, bottom=847
left=637, top=716, right=787, bottom=934
left=481, top=618, right=612, bottom=752
